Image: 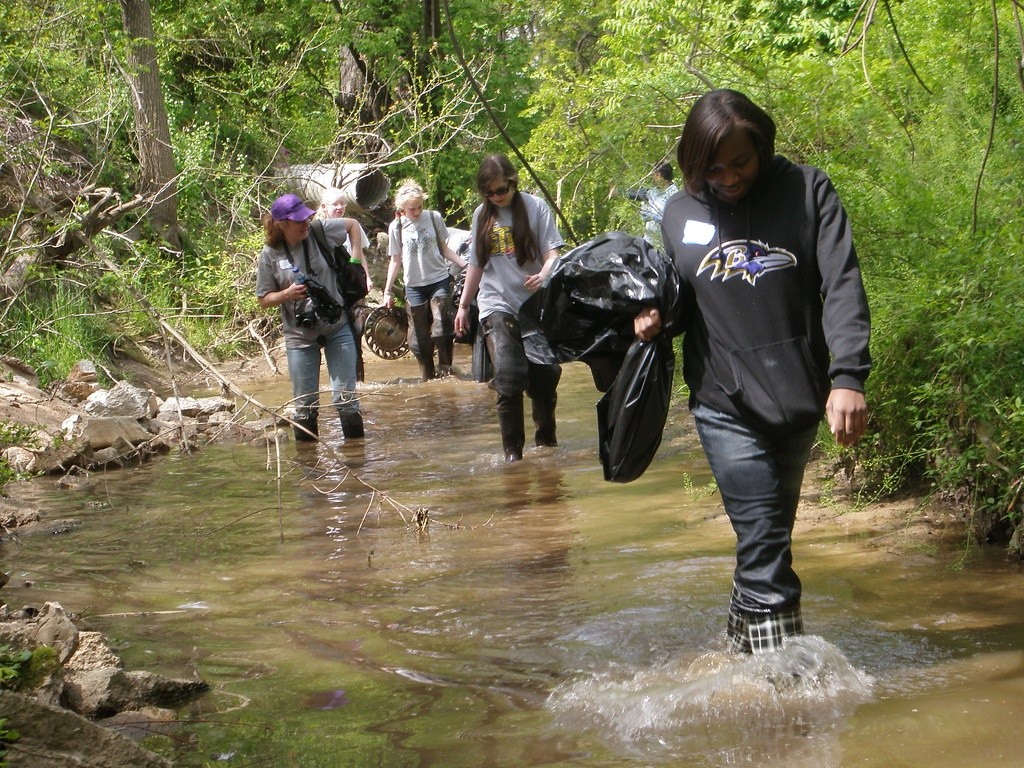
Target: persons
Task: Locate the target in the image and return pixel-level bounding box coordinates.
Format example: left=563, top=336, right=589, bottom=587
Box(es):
left=381, top=183, right=469, bottom=380
left=612, top=164, right=680, bottom=244
left=257, top=196, right=365, bottom=440
left=311, top=188, right=373, bottom=382
left=454, top=154, right=564, bottom=459
left=635, top=90, right=870, bottom=655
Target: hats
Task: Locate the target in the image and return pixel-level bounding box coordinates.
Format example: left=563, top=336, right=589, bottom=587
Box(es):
left=271, top=194, right=316, bottom=221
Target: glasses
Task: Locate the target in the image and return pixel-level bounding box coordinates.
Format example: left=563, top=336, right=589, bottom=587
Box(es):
left=484, top=180, right=512, bottom=198
left=327, top=203, right=348, bottom=208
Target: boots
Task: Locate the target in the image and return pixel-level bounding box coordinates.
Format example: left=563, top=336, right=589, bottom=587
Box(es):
left=724, top=581, right=806, bottom=676
left=414, top=355, right=433, bottom=382
left=433, top=338, right=454, bottom=378
left=290, top=418, right=319, bottom=443
left=339, top=412, right=365, bottom=440
left=495, top=410, right=525, bottom=464
left=530, top=395, right=558, bottom=447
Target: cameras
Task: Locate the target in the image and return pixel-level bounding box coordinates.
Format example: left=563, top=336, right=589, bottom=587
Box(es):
left=293, top=293, right=319, bottom=328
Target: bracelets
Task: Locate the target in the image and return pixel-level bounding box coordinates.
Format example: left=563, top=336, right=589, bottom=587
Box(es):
left=459, top=305, right=470, bottom=310
left=349, top=258, right=361, bottom=263
left=386, top=292, right=395, bottom=297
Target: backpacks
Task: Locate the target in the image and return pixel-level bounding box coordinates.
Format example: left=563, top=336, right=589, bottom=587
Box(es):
left=309, top=219, right=368, bottom=307
